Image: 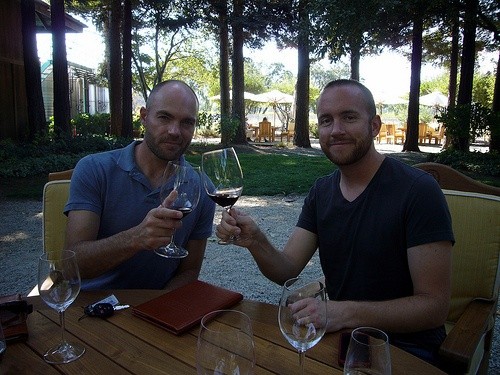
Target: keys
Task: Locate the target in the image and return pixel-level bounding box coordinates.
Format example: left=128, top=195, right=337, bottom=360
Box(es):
left=79, top=294, right=130, bottom=320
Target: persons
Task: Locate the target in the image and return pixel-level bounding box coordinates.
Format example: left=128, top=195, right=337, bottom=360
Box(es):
left=245, top=116, right=269, bottom=142
left=216, top=80, right=456, bottom=372
left=64, top=79, right=216, bottom=295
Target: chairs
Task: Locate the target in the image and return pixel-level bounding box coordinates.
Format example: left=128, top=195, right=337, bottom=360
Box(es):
left=285, top=162, right=500, bottom=375
left=43, top=169, right=73, bottom=270
left=250, top=122, right=445, bottom=146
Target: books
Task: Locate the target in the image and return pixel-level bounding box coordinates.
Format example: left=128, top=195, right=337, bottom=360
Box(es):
left=0, top=295, right=29, bottom=344
left=130, top=279, right=243, bottom=336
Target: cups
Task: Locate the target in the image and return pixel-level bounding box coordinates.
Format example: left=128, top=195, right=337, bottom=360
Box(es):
left=196, top=309, right=255, bottom=375
left=344, top=326, right=391, bottom=375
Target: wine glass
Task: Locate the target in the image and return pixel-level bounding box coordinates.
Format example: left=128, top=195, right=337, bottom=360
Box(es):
left=202, top=147, right=244, bottom=246
left=38, top=250, right=86, bottom=365
left=277, top=277, right=328, bottom=375
left=153, top=160, right=202, bottom=258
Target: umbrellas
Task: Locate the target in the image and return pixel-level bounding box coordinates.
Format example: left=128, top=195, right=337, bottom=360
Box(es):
left=419, top=91, right=448, bottom=106
left=250, top=90, right=296, bottom=134
left=374, top=92, right=410, bottom=104
left=207, top=89, right=256, bottom=102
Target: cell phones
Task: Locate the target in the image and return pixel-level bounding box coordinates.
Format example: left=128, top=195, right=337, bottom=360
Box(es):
left=338, top=332, right=372, bottom=369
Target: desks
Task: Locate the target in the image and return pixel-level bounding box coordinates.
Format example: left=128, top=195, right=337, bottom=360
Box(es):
left=0, top=289, right=441, bottom=375
left=255, top=127, right=277, bottom=142
